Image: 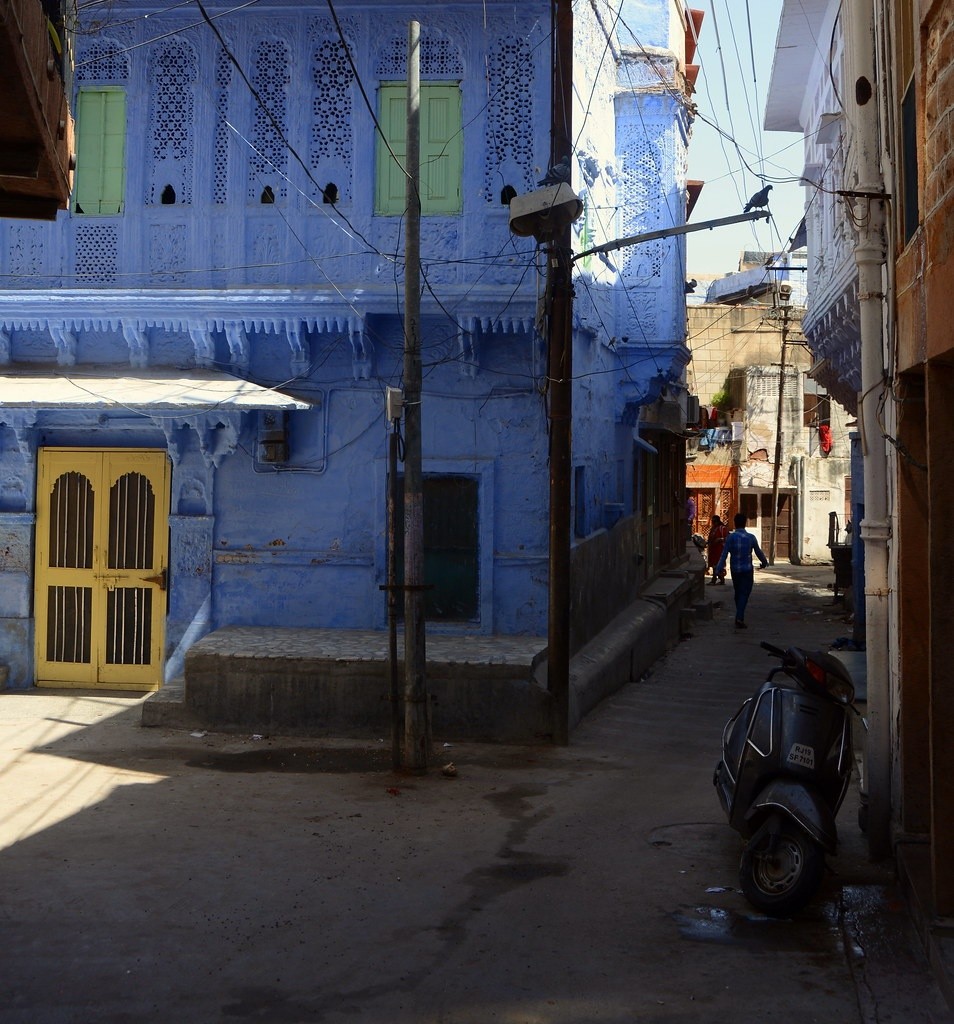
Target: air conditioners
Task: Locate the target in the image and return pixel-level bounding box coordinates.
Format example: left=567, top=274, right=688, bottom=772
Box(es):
left=686, top=395, right=699, bottom=424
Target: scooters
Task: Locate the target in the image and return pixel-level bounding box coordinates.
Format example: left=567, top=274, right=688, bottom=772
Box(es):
left=713, top=641, right=868, bottom=921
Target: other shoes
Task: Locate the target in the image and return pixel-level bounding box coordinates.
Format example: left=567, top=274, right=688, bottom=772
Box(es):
left=716, top=580, right=725, bottom=585
left=735, top=621, right=748, bottom=629
left=707, top=580, right=715, bottom=585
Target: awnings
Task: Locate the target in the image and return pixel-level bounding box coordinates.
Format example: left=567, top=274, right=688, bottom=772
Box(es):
left=0, top=367, right=322, bottom=409
left=800, top=163, right=821, bottom=187
left=815, top=113, right=840, bottom=143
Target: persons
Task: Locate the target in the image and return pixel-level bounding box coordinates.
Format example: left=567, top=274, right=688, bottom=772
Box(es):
left=706, top=515, right=729, bottom=586
left=717, top=514, right=768, bottom=629
left=685, top=488, right=695, bottom=540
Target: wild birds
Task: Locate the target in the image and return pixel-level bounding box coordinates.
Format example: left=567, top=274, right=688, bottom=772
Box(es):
left=688, top=253, right=775, bottom=289
left=435, top=742, right=458, bottom=773
left=537, top=156, right=571, bottom=187
left=743, top=185, right=774, bottom=212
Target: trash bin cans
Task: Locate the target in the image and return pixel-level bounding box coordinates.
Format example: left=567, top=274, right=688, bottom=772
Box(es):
left=831, top=546, right=852, bottom=588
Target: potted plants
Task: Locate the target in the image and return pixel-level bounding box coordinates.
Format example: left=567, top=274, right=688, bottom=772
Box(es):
left=712, top=384, right=732, bottom=426
